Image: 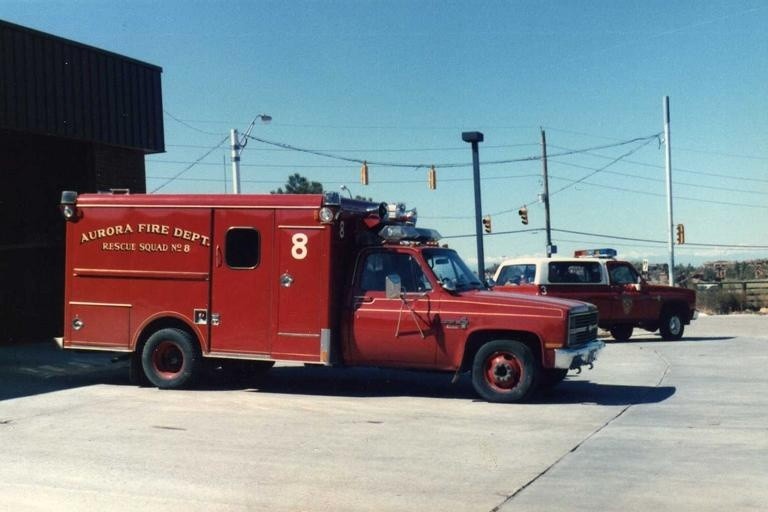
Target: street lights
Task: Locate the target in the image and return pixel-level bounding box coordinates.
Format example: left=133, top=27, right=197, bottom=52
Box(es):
left=231, top=114, right=272, bottom=194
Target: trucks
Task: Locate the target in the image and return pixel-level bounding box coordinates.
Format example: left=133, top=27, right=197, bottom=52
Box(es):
left=61, top=192, right=604, bottom=403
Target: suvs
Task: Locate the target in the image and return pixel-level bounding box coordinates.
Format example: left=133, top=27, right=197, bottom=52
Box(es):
left=493, top=249, right=696, bottom=340
